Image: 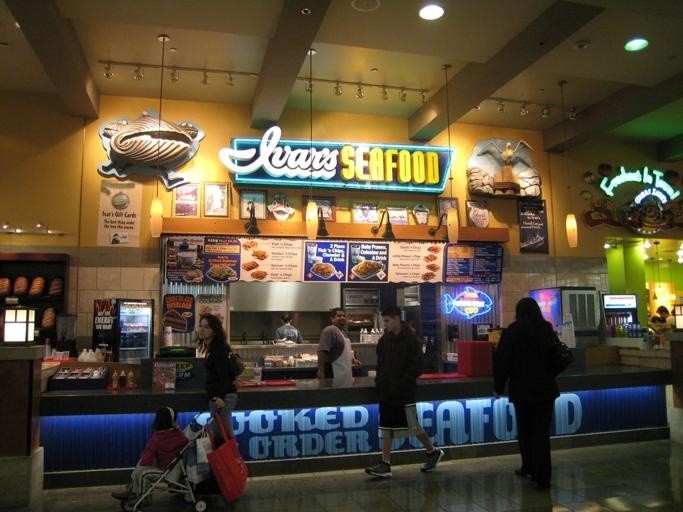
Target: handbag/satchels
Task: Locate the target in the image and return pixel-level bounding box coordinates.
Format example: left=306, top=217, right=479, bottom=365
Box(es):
left=196, top=431, right=216, bottom=475
left=184, top=444, right=206, bottom=484
left=204, top=411, right=250, bottom=503
left=540, top=338, right=572, bottom=376
left=227, top=352, right=246, bottom=381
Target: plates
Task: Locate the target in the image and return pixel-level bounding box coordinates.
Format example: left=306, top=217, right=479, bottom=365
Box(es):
left=178, top=264, right=238, bottom=283
left=309, top=262, right=345, bottom=280
left=351, top=260, right=387, bottom=279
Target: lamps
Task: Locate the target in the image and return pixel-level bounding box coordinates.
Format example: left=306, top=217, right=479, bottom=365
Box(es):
left=554, top=79, right=578, bottom=250
left=317, top=206, right=330, bottom=237
left=243, top=200, right=262, bottom=235
left=101, top=61, right=235, bottom=88
left=0, top=303, right=41, bottom=347
left=370, top=209, right=397, bottom=242
left=428, top=211, right=451, bottom=244
left=473, top=96, right=577, bottom=121
left=440, top=62, right=463, bottom=247
left=304, top=78, right=431, bottom=104
left=149, top=35, right=171, bottom=239
left=304, top=47, right=318, bottom=242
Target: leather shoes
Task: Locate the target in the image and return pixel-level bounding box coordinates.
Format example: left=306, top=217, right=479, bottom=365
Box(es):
left=515, top=464, right=552, bottom=490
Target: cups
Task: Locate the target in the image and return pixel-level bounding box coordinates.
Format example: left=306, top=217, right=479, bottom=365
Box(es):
left=162, top=326, right=173, bottom=348
left=349, top=245, right=358, bottom=264
left=44, top=337, right=70, bottom=361
left=305, top=243, right=316, bottom=264
left=251, top=367, right=262, bottom=384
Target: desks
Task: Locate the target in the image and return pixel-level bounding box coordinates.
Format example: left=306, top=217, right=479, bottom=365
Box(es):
left=258, top=361, right=319, bottom=381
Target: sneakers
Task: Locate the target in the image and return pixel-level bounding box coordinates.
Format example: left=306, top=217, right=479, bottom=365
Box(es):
left=364, top=458, right=393, bottom=478
left=111, top=486, right=153, bottom=508
left=420, top=447, right=445, bottom=474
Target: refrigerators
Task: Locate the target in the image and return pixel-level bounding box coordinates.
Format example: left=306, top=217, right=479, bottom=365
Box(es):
left=92, top=299, right=155, bottom=365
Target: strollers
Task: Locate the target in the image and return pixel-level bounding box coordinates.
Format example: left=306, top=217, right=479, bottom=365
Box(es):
left=119, top=397, right=224, bottom=511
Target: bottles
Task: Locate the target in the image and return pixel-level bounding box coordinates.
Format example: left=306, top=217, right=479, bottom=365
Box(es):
left=358, top=328, right=388, bottom=344
left=118, top=321, right=149, bottom=363
left=264, top=353, right=318, bottom=368
left=78, top=348, right=103, bottom=363
left=607, top=322, right=644, bottom=338
left=110, top=369, right=136, bottom=391
left=653, top=334, right=665, bottom=349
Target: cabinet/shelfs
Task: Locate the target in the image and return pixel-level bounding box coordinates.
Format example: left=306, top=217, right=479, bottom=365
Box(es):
left=0, top=252, right=70, bottom=344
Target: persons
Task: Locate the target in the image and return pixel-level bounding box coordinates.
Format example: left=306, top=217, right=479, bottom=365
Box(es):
left=656, top=305, right=675, bottom=327
left=315, top=306, right=362, bottom=379
left=273, top=311, right=304, bottom=343
left=196, top=312, right=237, bottom=498
left=492, top=297, right=560, bottom=490
left=127, top=405, right=189, bottom=508
left=363, top=305, right=444, bottom=478
